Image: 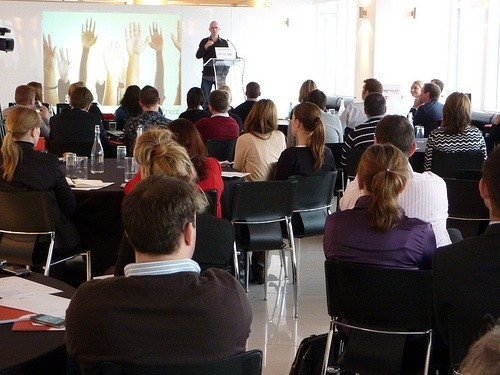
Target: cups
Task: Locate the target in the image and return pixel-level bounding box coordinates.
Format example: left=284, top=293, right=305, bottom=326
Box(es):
left=33, top=100, right=42, bottom=113
left=417, top=126, right=424, bottom=143
left=109, top=122, right=116, bottom=132
left=329, top=109, right=335, bottom=115
left=124, top=157, right=137, bottom=183
left=117, top=146, right=126, bottom=168
left=66, top=153, right=77, bottom=178
left=76, top=157, right=88, bottom=180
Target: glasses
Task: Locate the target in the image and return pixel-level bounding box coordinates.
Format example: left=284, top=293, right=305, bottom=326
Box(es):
left=288, top=118, right=297, bottom=123
left=211, top=27, right=219, bottom=29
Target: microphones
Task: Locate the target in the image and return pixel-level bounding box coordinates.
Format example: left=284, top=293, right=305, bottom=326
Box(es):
left=227, top=39, right=240, bottom=59
left=0, top=28, right=11, bottom=33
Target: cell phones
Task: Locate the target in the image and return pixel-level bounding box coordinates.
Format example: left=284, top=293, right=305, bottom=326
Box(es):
left=31, top=314, right=65, bottom=328
left=2, top=265, right=32, bottom=276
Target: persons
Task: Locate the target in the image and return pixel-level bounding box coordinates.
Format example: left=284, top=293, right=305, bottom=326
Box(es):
left=406, top=79, right=488, bottom=170
left=196, top=21, right=228, bottom=107
left=107, top=118, right=234, bottom=276
left=323, top=116, right=452, bottom=280
left=65, top=174, right=253, bottom=375
left=0, top=81, right=53, bottom=138
left=179, top=80, right=344, bottom=286
left=115, top=84, right=172, bottom=155
left=47, top=81, right=109, bottom=157
left=44, top=17, right=181, bottom=105
left=340, top=79, right=387, bottom=170
left=0, top=106, right=82, bottom=279
left=433, top=146, right=500, bottom=375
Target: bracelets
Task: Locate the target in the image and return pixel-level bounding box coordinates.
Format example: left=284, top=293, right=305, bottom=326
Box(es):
left=45, top=86, right=57, bottom=90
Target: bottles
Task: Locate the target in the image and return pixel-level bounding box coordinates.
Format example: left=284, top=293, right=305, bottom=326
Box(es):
left=339, top=100, right=345, bottom=117
left=288, top=102, right=293, bottom=121
left=132, top=125, right=142, bottom=174
left=90, top=125, right=104, bottom=174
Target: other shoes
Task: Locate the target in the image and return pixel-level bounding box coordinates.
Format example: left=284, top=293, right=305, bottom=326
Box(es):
left=249, top=264, right=265, bottom=284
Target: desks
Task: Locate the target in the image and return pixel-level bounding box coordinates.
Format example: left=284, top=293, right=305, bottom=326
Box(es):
left=0, top=265, right=77, bottom=375
left=53, top=158, right=244, bottom=272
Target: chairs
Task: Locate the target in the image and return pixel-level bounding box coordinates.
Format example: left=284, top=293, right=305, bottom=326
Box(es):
left=0, top=139, right=491, bottom=375
